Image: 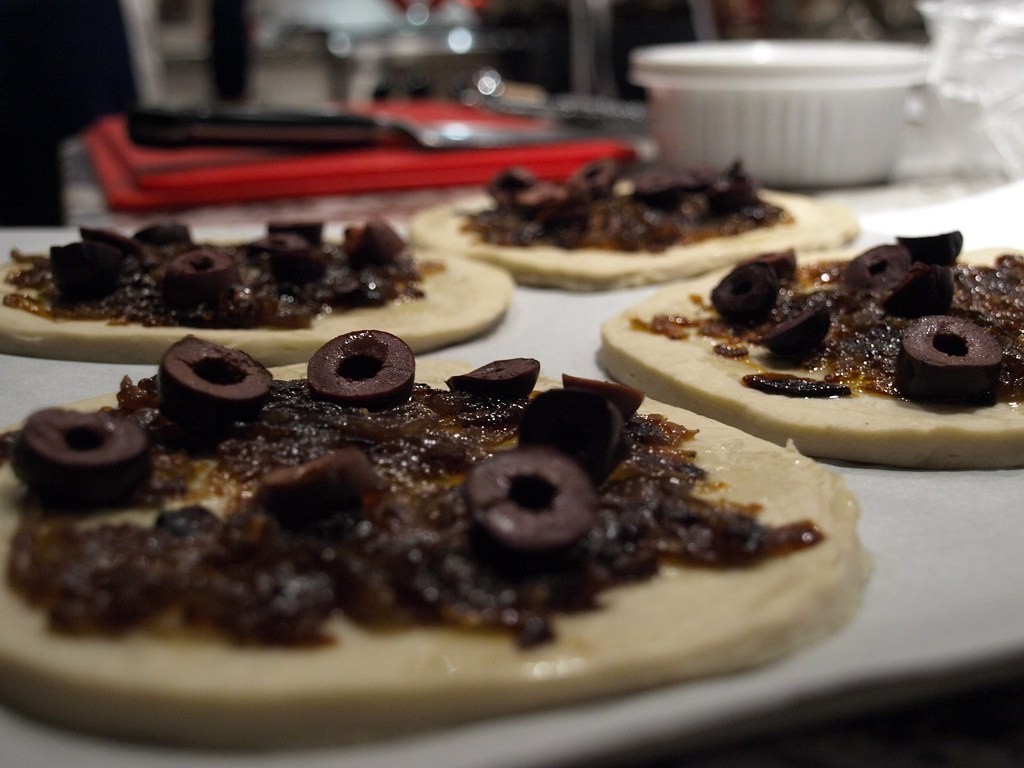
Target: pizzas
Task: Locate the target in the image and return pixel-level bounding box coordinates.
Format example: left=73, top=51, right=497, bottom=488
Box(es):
left=0, top=157, right=1024, bottom=750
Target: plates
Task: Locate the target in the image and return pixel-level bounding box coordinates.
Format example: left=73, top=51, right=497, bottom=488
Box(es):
left=0, top=182, right=1024, bottom=768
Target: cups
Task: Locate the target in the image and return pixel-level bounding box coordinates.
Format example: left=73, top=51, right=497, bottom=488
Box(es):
left=914, top=0, right=1024, bottom=179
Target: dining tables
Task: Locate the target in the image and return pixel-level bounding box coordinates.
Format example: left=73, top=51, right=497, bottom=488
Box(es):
left=0, top=203, right=1024, bottom=768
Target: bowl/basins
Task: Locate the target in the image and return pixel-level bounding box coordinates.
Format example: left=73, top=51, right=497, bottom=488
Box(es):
left=630, top=36, right=944, bottom=190
